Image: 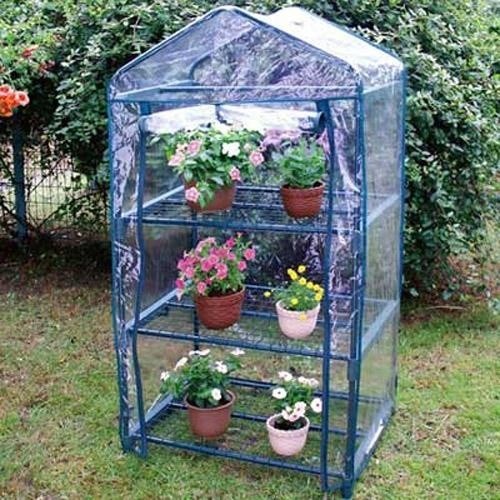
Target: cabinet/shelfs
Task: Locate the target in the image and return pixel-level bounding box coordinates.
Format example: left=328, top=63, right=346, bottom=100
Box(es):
left=120, top=186, right=402, bottom=495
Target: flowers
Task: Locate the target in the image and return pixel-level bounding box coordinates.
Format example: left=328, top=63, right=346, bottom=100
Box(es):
left=248, top=125, right=329, bottom=188
left=158, top=345, right=249, bottom=406
left=176, top=230, right=254, bottom=301
left=263, top=265, right=325, bottom=321
left=146, top=122, right=255, bottom=206
left=271, top=371, right=323, bottom=431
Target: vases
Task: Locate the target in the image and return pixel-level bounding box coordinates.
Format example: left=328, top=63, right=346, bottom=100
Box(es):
left=195, top=286, right=246, bottom=329
left=275, top=299, right=320, bottom=340
left=280, top=182, right=326, bottom=217
left=266, top=413, right=310, bottom=456
left=184, top=179, right=238, bottom=214
left=184, top=391, right=236, bottom=438
